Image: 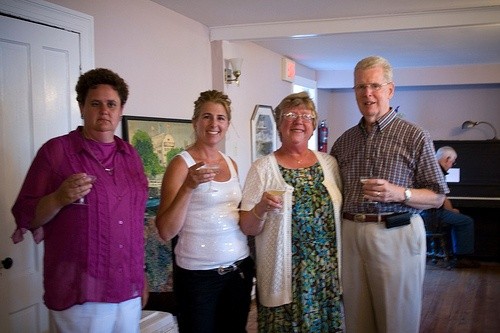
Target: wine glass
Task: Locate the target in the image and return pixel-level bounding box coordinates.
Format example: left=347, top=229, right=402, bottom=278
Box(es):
left=71, top=175, right=97, bottom=206
left=199, top=161, right=221, bottom=194
left=359, top=175, right=380, bottom=204
left=267, top=185, right=287, bottom=215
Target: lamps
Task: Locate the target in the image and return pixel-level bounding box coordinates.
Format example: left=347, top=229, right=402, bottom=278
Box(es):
left=462, top=120, right=497, bottom=139
left=226, top=58, right=244, bottom=85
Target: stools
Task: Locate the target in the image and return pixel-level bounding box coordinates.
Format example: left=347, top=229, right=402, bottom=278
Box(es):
left=421, top=213, right=457, bottom=270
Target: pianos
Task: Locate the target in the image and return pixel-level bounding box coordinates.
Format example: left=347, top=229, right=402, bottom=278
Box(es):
left=432, top=138, right=500, bottom=263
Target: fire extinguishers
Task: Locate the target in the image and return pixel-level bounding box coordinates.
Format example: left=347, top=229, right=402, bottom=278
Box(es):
left=318, top=120, right=328, bottom=152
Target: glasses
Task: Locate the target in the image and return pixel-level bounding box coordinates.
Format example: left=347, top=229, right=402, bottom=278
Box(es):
left=276, top=112, right=314, bottom=120
left=351, top=81, right=391, bottom=90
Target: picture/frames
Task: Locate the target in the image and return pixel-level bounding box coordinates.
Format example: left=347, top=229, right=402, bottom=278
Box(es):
left=250, top=105, right=277, bottom=164
left=122, top=115, right=197, bottom=187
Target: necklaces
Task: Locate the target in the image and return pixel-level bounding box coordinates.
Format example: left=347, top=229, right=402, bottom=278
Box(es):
left=296, top=159, right=304, bottom=164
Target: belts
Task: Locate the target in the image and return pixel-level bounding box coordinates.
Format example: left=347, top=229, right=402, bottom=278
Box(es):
left=344, top=211, right=395, bottom=223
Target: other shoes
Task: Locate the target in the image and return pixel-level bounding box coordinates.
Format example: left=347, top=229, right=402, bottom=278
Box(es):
left=456, top=260, right=480, bottom=268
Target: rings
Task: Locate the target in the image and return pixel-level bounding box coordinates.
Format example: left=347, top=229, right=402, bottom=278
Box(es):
left=377, top=191, right=381, bottom=197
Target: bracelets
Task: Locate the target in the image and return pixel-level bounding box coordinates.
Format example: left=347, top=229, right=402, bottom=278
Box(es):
left=252, top=203, right=266, bottom=220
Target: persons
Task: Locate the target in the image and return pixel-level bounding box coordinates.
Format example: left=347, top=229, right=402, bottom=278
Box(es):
left=330, top=55, right=449, bottom=333
left=419, top=145, right=480, bottom=268
left=155, top=89, right=254, bottom=332
left=10, top=67, right=150, bottom=333
left=240, top=91, right=344, bottom=333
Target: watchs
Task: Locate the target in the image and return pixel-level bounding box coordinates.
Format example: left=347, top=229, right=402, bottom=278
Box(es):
left=401, top=187, right=412, bottom=204
